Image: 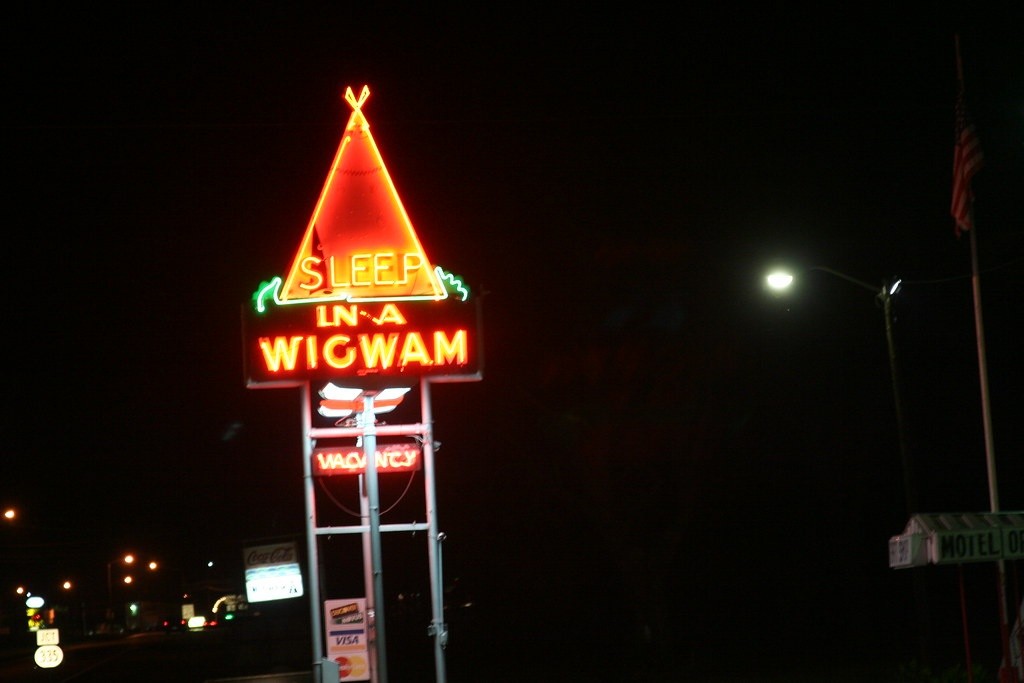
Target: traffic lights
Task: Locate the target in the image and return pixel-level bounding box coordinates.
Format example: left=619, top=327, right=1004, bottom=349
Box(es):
left=128, top=603, right=138, bottom=616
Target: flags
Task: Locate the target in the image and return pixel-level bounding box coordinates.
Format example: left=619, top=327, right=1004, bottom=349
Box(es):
left=946, top=51, right=994, bottom=240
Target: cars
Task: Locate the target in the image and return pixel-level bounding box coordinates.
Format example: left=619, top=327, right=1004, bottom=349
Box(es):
left=163, top=616, right=188, bottom=635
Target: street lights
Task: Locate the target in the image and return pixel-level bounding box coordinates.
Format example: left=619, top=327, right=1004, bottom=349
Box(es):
left=107, top=554, right=133, bottom=637
left=64, top=575, right=87, bottom=637
left=758, top=263, right=914, bottom=512
